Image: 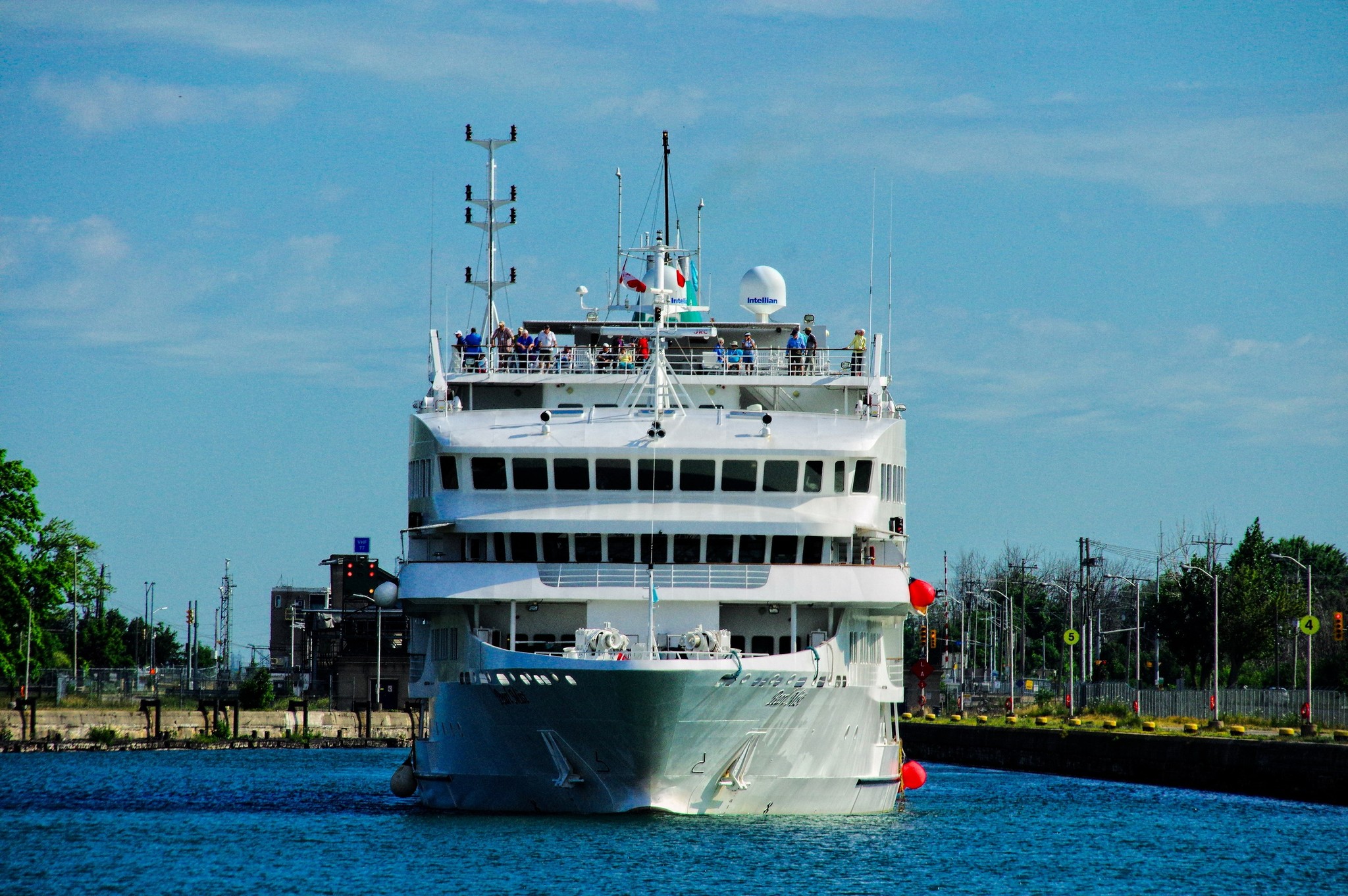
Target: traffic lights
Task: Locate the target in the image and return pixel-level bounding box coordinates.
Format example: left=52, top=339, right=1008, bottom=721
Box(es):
left=343, top=557, right=378, bottom=598
left=920, top=626, right=926, bottom=646
left=1334, top=612, right=1343, bottom=641
left=930, top=630, right=936, bottom=648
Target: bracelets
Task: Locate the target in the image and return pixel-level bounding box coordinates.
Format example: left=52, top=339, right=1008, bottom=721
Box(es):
left=555, top=344, right=556, bottom=345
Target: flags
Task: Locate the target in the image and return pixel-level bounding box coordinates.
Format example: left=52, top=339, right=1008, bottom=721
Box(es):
left=619, top=266, right=646, bottom=293
left=691, top=260, right=698, bottom=292
left=676, top=262, right=685, bottom=288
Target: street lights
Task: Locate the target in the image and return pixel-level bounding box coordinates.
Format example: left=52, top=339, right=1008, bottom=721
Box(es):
left=1155, top=543, right=1200, bottom=690
left=1033, top=607, right=1067, bottom=697
left=1271, top=553, right=1312, bottom=725
left=1179, top=564, right=1218, bottom=720
left=144, top=581, right=167, bottom=689
left=938, top=588, right=1014, bottom=714
left=74, top=546, right=78, bottom=696
left=1103, top=574, right=1141, bottom=717
left=25, top=587, right=35, bottom=710
left=1039, top=582, right=1074, bottom=717
left=1100, top=612, right=1133, bottom=684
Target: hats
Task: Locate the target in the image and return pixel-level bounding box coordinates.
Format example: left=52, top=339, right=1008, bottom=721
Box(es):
left=498, top=321, right=505, bottom=326
left=730, top=341, right=738, bottom=347
left=803, top=327, right=811, bottom=331
left=790, top=330, right=797, bottom=335
left=454, top=330, right=461, bottom=335
left=517, top=327, right=523, bottom=331
left=603, top=343, right=609, bottom=347
left=744, top=332, right=751, bottom=336
left=544, top=325, right=550, bottom=329
left=565, top=345, right=571, bottom=349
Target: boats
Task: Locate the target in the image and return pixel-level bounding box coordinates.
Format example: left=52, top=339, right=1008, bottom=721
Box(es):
left=396, top=121, right=911, bottom=819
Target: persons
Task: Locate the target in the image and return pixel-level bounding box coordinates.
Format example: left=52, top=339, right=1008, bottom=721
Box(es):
left=611, top=335, right=625, bottom=374
left=789, top=326, right=817, bottom=376
left=492, top=321, right=557, bottom=374
left=713, top=337, right=725, bottom=364
left=724, top=341, right=743, bottom=375
left=617, top=347, right=634, bottom=374
left=553, top=344, right=578, bottom=374
left=597, top=343, right=614, bottom=374
left=630, top=337, right=650, bottom=375
left=454, top=328, right=488, bottom=373
left=785, top=330, right=806, bottom=376
left=842, top=328, right=867, bottom=377
left=741, top=332, right=755, bottom=375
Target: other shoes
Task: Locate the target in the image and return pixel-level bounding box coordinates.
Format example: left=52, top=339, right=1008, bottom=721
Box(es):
left=603, top=370, right=608, bottom=374
